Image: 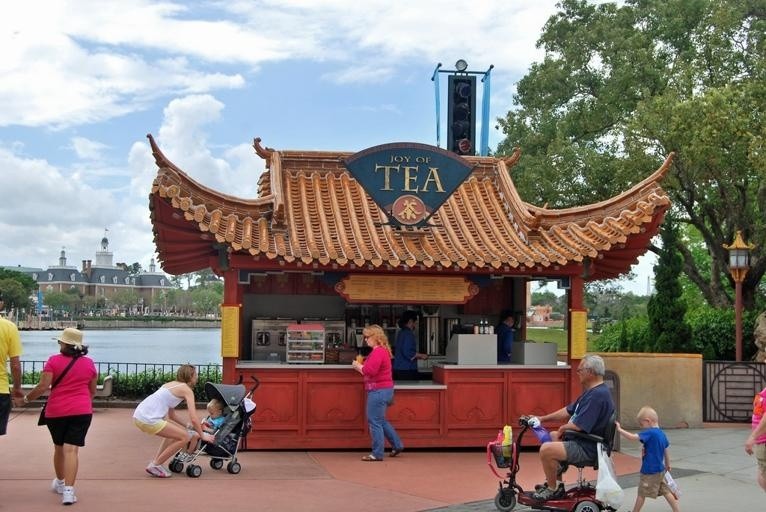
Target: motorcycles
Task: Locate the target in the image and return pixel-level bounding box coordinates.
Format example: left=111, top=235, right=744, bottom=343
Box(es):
left=487, top=412, right=619, bottom=512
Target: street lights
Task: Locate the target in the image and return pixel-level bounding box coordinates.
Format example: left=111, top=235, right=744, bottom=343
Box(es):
left=721, top=228, right=760, bottom=363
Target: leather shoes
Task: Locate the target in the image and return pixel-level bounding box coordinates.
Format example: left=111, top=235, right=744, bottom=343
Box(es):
left=362, top=454, right=383, bottom=462
left=390, top=445, right=404, bottom=457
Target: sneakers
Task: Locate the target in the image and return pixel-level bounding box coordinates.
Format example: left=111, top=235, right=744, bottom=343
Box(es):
left=535, top=481, right=548, bottom=492
left=146, top=463, right=172, bottom=478
left=52, top=477, right=66, bottom=494
left=532, top=482, right=566, bottom=502
left=185, top=455, right=196, bottom=463
left=178, top=453, right=188, bottom=462
left=63, top=486, right=74, bottom=504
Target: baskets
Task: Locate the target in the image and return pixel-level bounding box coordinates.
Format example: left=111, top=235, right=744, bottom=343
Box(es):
left=491, top=442, right=513, bottom=468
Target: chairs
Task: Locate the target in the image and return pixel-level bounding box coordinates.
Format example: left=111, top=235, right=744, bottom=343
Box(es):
left=562, top=412, right=621, bottom=471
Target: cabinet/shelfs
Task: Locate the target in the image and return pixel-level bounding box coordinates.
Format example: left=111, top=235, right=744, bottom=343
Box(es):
left=286, top=325, right=326, bottom=363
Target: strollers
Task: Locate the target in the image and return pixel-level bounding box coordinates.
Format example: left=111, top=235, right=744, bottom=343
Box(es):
left=168, top=373, right=262, bottom=477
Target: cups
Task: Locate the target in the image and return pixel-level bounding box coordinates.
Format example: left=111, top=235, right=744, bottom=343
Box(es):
left=473, top=323, right=480, bottom=334
left=489, top=323, right=494, bottom=334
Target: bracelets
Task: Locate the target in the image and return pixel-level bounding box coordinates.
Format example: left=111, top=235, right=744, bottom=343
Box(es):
left=23, top=395, right=30, bottom=404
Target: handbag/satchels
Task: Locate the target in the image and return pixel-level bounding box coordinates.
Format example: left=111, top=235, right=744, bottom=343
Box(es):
left=38, top=402, right=47, bottom=426
left=595, top=442, right=625, bottom=510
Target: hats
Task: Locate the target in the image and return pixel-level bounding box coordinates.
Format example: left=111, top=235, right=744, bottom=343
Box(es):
left=60, top=327, right=83, bottom=350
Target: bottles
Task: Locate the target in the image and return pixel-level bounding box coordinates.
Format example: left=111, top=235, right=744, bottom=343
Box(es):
left=497, top=424, right=513, bottom=458
left=479, top=317, right=489, bottom=334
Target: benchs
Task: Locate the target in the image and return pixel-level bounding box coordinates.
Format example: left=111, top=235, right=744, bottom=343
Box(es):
left=9, top=375, right=114, bottom=398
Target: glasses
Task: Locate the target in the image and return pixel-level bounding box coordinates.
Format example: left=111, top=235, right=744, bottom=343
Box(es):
left=365, top=334, right=374, bottom=340
left=58, top=340, right=61, bottom=344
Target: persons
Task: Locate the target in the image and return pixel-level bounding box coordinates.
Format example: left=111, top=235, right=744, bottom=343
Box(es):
left=351, top=324, right=404, bottom=461
left=0, top=290, right=25, bottom=437
left=133, top=363, right=216, bottom=478
left=176, top=398, right=227, bottom=464
left=10, top=327, right=97, bottom=505
left=392, top=309, right=429, bottom=380
left=527, top=353, right=617, bottom=502
left=745, top=385, right=766, bottom=493
left=493, top=308, right=518, bottom=361
left=614, top=405, right=682, bottom=512
